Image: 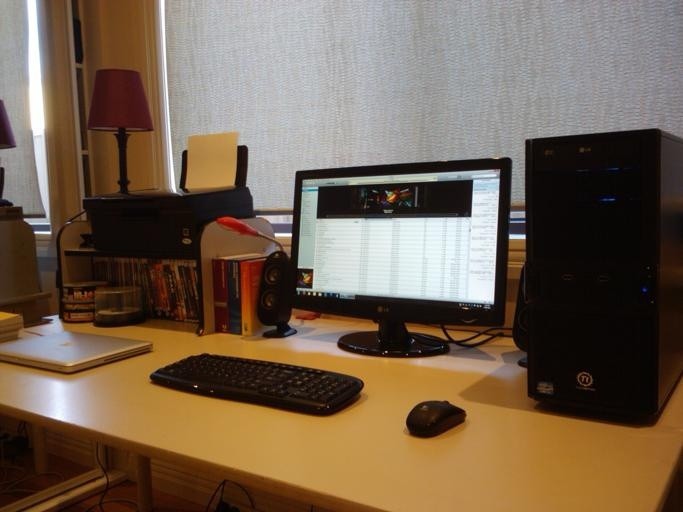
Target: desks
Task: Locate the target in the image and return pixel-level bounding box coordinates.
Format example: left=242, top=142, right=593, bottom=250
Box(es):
left=0, top=291, right=683, bottom=511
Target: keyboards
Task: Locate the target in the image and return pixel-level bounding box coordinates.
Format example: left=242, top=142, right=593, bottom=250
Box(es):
left=149, top=353, right=363, bottom=416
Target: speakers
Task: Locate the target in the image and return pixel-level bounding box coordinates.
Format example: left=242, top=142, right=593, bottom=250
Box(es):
left=254, top=249, right=297, bottom=340
left=512, top=266, right=530, bottom=369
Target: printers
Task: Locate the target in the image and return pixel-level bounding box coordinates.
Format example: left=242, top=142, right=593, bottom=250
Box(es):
left=83, top=131, right=256, bottom=260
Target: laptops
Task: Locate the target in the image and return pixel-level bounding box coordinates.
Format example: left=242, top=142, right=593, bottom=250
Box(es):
left=1, top=331, right=152, bottom=375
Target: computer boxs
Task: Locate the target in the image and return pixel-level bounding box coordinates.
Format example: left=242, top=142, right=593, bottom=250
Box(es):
left=524, top=129, right=682, bottom=430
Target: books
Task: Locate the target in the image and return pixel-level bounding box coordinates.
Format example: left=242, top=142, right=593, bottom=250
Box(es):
left=211, top=251, right=268, bottom=337
left=0, top=312, right=23, bottom=343
left=95, top=257, right=200, bottom=323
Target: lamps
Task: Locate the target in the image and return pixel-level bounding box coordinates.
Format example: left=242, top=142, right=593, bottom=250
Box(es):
left=87, top=68, right=153, bottom=199
left=0, top=98, right=16, bottom=208
left=216, top=215, right=284, bottom=254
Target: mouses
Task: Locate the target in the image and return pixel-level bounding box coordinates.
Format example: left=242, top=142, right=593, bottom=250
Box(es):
left=406, top=399, right=467, bottom=438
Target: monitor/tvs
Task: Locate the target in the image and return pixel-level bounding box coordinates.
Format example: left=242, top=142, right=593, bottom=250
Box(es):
left=289, top=157, right=516, bottom=357
